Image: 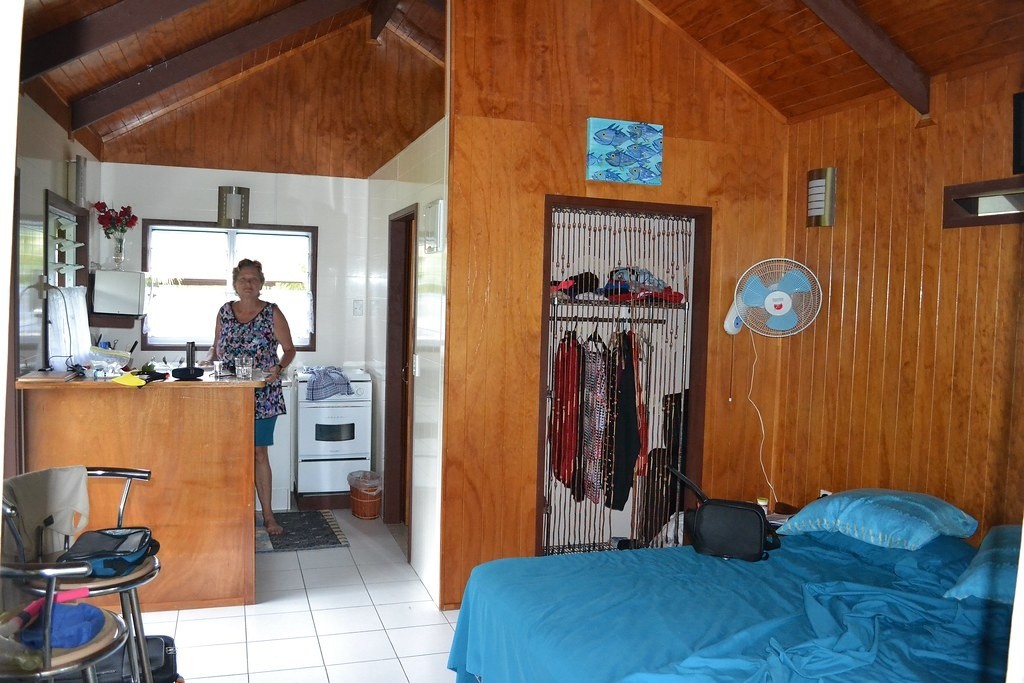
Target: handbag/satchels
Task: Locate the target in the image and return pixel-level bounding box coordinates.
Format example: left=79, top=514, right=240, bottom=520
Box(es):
left=56, top=526, right=160, bottom=578
left=685, top=498, right=781, bottom=562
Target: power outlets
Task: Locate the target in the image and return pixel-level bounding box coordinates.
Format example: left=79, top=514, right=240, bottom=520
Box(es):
left=40, top=275, right=47, bottom=299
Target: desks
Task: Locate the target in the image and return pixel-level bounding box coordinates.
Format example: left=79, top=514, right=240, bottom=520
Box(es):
left=16, top=368, right=264, bottom=614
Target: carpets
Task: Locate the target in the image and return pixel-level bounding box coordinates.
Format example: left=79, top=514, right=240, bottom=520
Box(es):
left=255, top=510, right=349, bottom=553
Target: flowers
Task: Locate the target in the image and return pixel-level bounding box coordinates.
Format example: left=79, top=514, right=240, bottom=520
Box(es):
left=94, top=200, right=138, bottom=252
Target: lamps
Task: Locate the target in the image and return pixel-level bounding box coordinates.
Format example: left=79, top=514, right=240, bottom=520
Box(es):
left=217, top=186, right=250, bottom=228
left=51, top=217, right=85, bottom=274
left=806, top=167, right=834, bottom=226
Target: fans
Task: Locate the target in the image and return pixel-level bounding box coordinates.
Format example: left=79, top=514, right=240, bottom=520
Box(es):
left=724, top=257, right=823, bottom=402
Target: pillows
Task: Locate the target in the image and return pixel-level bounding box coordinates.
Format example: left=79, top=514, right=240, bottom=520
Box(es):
left=943, top=525, right=1022, bottom=605
left=805, top=531, right=976, bottom=574
left=775, top=488, right=978, bottom=550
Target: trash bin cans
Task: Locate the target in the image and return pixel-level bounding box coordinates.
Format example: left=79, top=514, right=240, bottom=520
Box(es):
left=348, top=471, right=381, bottom=520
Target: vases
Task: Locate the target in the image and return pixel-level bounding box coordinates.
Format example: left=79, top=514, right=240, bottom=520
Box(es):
left=112, top=232, right=125, bottom=270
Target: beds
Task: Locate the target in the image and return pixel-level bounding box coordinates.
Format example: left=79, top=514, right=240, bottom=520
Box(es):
left=448, top=536, right=1014, bottom=683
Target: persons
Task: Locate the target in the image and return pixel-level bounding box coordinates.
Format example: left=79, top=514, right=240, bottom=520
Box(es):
left=200, top=259, right=295, bottom=533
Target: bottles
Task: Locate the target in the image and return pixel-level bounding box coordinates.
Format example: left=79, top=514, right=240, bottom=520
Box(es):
left=757, top=498, right=767, bottom=516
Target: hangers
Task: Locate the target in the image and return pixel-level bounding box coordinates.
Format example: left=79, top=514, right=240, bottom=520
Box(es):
left=561, top=316, right=651, bottom=361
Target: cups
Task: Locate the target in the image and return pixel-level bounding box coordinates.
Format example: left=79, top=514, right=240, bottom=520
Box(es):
left=213, top=361, right=223, bottom=376
left=235, top=358, right=252, bottom=378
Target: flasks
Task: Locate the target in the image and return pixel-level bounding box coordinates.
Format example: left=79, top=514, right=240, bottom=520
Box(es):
left=187, top=342, right=195, bottom=374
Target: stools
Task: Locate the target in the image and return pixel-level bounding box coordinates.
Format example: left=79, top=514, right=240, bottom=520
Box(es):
left=0, top=467, right=161, bottom=683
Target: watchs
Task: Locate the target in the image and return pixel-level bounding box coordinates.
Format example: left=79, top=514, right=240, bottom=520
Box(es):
left=276, top=363, right=285, bottom=372
